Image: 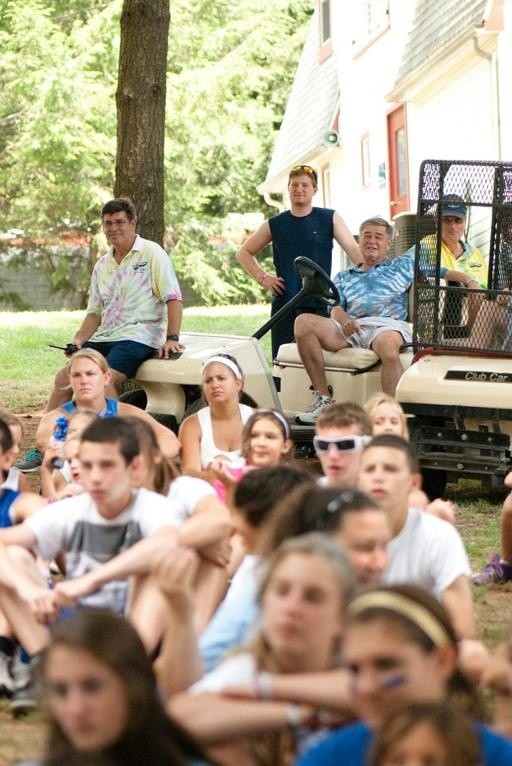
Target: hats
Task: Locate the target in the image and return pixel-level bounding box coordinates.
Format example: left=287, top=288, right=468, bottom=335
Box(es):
left=440, top=195, right=467, bottom=219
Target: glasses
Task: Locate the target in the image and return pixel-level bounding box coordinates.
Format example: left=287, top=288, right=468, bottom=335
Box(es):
left=313, top=435, right=369, bottom=453
left=291, top=165, right=318, bottom=185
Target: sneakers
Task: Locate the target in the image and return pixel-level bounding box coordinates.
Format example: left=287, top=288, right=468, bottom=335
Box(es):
left=13, top=449, right=42, bottom=472
left=474, top=554, right=509, bottom=586
left=1, top=641, right=43, bottom=716
left=296, top=395, right=335, bottom=426
